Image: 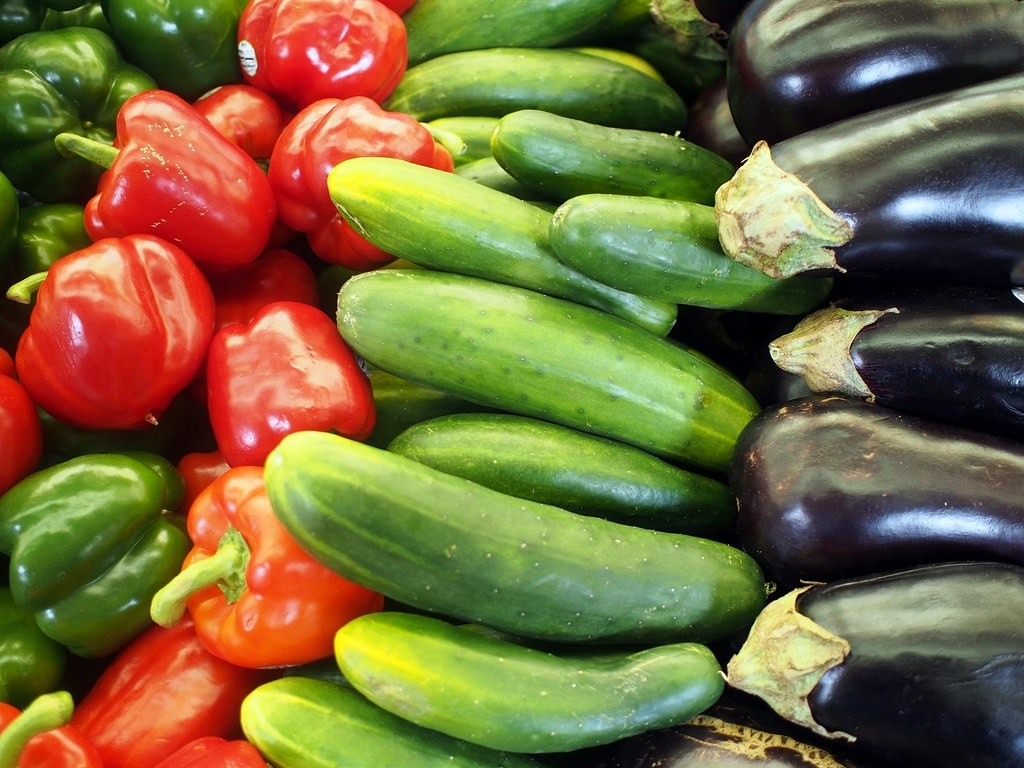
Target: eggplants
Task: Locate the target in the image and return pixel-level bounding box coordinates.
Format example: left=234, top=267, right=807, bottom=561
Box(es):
left=646, top=0, right=1024, bottom=768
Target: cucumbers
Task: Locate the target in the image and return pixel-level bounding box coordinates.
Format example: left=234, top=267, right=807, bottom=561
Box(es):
left=239, top=1, right=834, bottom=768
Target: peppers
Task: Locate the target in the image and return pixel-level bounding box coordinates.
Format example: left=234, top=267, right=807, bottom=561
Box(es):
left=0, top=0, right=456, bottom=768
